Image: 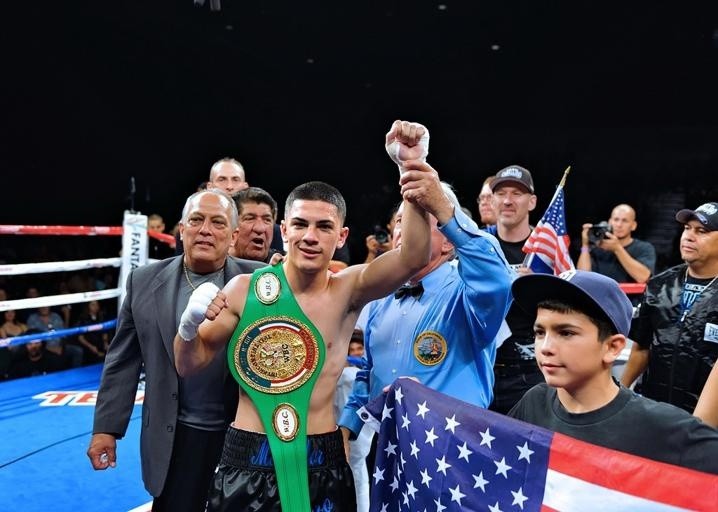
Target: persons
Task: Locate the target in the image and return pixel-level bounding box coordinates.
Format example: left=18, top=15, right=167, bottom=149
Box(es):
left=497, top=270, right=717, bottom=478
left=337, top=158, right=516, bottom=512
left=694, top=357, right=718, bottom=432
left=0, top=157, right=657, bottom=382
left=623, top=200, right=717, bottom=412
left=170, top=115, right=433, bottom=512
left=85, top=186, right=265, bottom=512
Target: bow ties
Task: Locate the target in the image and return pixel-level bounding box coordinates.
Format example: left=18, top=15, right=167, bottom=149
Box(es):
left=395, top=281, right=424, bottom=301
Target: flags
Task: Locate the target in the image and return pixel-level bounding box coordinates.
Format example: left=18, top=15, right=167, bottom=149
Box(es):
left=355, top=372, right=718, bottom=512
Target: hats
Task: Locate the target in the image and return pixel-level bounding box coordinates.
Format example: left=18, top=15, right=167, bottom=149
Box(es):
left=676, top=202, right=717, bottom=230
left=512, top=271, right=633, bottom=337
left=491, top=165, right=536, bottom=194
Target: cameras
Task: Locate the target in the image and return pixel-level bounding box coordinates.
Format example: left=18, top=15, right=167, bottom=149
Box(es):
left=588, top=221, right=613, bottom=243
left=373, top=225, right=389, bottom=244
